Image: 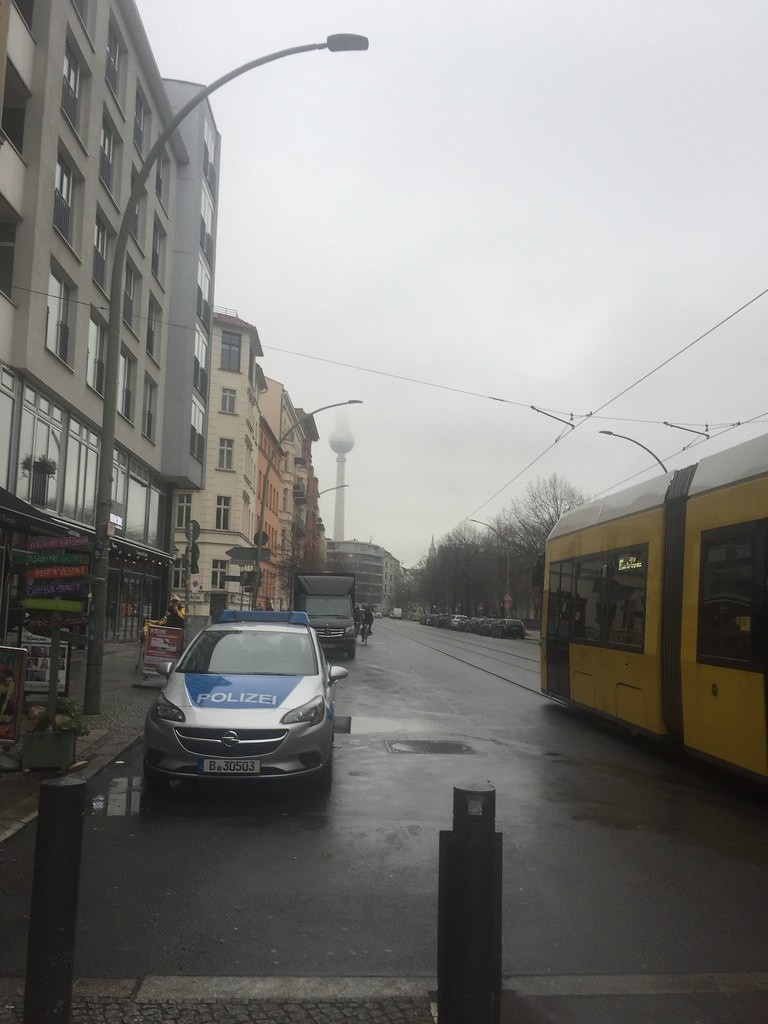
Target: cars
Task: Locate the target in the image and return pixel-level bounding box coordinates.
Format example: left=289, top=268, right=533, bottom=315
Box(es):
left=372, top=612, right=383, bottom=619
left=139, top=607, right=350, bottom=790
left=419, top=613, right=526, bottom=640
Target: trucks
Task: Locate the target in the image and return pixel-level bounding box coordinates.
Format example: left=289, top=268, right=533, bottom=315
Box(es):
left=389, top=608, right=402, bottom=620
left=291, top=571, right=356, bottom=658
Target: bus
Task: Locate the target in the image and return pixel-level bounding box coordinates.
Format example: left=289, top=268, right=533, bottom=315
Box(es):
left=539, top=413, right=768, bottom=787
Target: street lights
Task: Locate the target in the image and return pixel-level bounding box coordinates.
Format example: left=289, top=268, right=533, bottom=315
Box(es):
left=291, top=485, right=350, bottom=611
left=468, top=519, right=510, bottom=619
left=80, top=33, right=373, bottom=714
left=250, top=399, right=364, bottom=611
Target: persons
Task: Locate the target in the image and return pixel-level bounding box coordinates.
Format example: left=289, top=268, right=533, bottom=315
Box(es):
left=253, top=603, right=274, bottom=611
left=361, top=606, right=373, bottom=642
left=23, top=646, right=65, bottom=681
left=161, top=596, right=185, bottom=629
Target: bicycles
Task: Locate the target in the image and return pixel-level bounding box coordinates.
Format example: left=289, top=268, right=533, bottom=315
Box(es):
left=359, top=622, right=371, bottom=645
